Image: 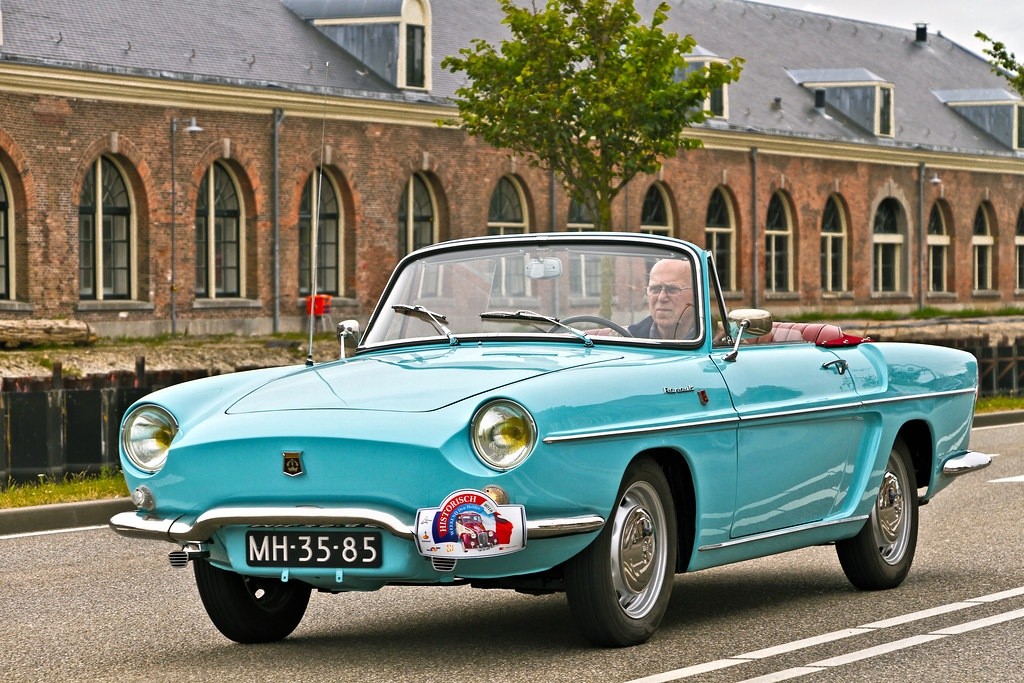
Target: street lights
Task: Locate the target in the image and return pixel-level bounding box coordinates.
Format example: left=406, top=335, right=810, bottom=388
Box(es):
left=918, top=161, right=942, bottom=309
left=170, top=116, right=204, bottom=337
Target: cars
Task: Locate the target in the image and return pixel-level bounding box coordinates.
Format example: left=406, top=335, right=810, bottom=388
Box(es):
left=108, top=231, right=993, bottom=648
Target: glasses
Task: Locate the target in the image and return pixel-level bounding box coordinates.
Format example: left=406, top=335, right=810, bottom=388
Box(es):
left=644, top=286, right=692, bottom=297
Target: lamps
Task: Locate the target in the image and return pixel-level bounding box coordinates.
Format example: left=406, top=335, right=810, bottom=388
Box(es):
left=182, top=117, right=204, bottom=138
left=929, top=172, right=942, bottom=185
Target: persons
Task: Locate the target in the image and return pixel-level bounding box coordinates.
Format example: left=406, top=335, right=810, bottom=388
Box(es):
left=623, top=259, right=698, bottom=340
